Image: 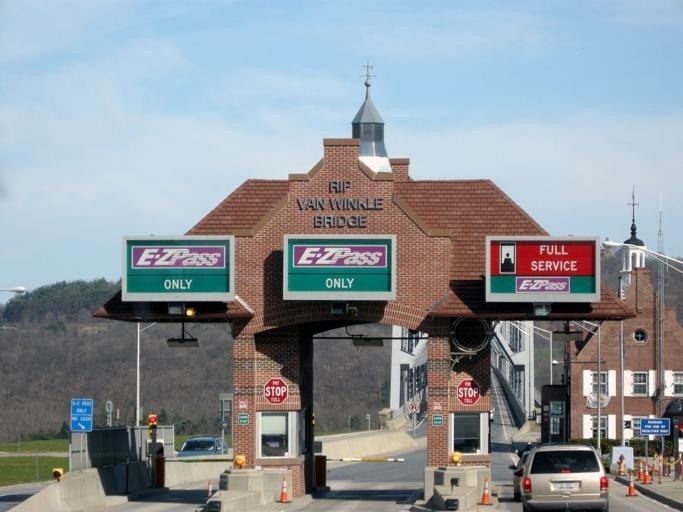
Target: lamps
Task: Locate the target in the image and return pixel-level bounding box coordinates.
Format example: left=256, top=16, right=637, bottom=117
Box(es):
left=167, top=322, right=200, bottom=348
left=345, top=325, right=385, bottom=348
left=553, top=320, right=583, bottom=342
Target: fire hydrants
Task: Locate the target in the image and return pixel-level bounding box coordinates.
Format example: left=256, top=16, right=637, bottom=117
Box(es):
left=617, top=454, right=626, bottom=476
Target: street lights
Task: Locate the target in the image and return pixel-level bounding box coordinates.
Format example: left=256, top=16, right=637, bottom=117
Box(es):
left=602, top=240, right=683, bottom=276
left=1, top=286, right=27, bottom=295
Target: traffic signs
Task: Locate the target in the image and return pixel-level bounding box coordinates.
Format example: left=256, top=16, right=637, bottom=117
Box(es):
left=456, top=378, right=482, bottom=407
left=262, top=377, right=289, bottom=406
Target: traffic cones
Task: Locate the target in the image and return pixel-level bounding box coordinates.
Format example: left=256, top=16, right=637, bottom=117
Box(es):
left=625, top=470, right=638, bottom=497
left=636, top=460, right=654, bottom=484
left=276, top=476, right=292, bottom=504
left=207, top=479, right=213, bottom=498
left=478, top=478, right=494, bottom=506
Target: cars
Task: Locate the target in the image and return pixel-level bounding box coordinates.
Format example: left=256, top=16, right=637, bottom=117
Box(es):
left=506, top=443, right=609, bottom=512
left=175, top=435, right=228, bottom=458
